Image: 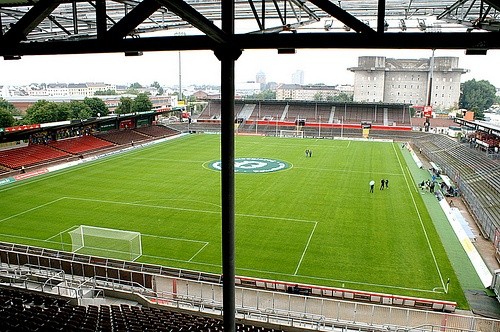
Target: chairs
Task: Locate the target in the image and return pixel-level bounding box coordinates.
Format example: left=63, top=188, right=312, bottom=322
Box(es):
left=0, top=284, right=284, bottom=332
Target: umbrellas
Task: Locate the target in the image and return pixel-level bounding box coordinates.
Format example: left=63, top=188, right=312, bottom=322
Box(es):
left=369, top=181, right=375, bottom=186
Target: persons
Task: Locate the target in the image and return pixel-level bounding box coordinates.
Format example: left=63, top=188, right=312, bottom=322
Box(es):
left=370, top=184, right=375, bottom=193
left=305, top=148, right=313, bottom=159
left=380, top=179, right=389, bottom=191
left=419, top=179, right=436, bottom=194
